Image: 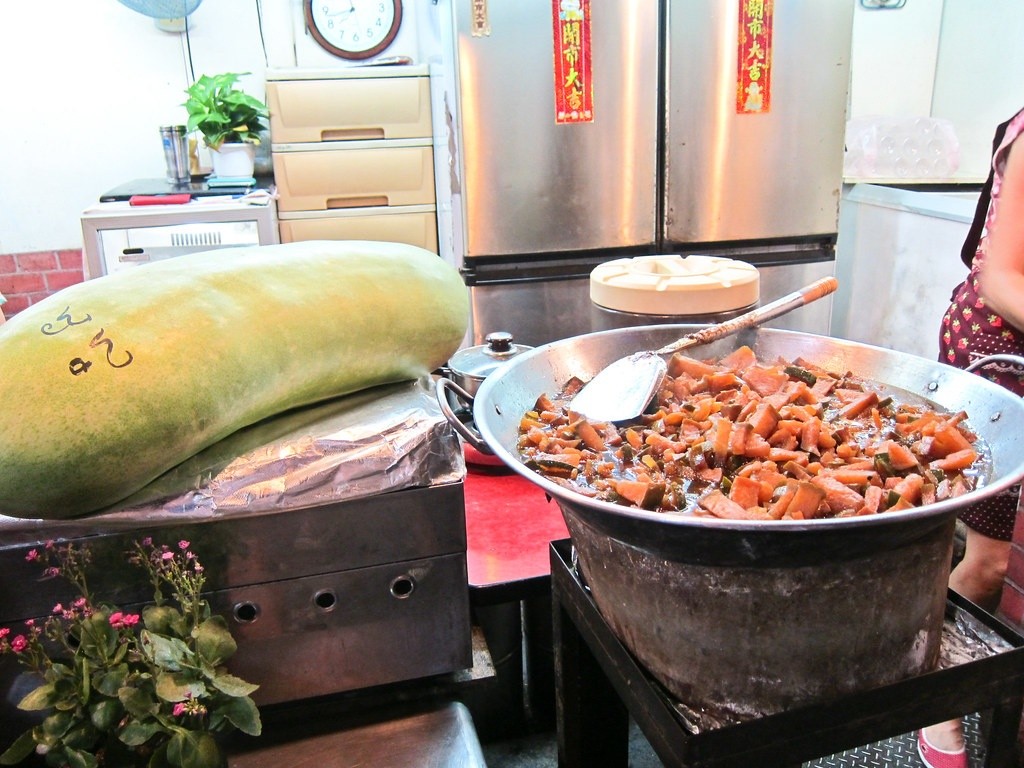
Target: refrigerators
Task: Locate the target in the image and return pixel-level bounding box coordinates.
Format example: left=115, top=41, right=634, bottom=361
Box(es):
left=413, top=0, right=856, bottom=350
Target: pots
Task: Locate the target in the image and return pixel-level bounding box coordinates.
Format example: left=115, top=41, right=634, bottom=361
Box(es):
left=435, top=322, right=1024, bottom=563
left=431, top=331, right=536, bottom=416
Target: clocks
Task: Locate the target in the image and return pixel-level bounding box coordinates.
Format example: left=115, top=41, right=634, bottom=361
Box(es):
left=302, top=0, right=402, bottom=61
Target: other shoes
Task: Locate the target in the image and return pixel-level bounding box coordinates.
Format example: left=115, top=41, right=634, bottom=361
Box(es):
left=917, top=723, right=968, bottom=768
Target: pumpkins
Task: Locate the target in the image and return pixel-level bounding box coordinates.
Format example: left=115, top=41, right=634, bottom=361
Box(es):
left=517, top=344, right=978, bottom=518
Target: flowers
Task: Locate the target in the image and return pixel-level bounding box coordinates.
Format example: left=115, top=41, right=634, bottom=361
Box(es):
left=0, top=538, right=261, bottom=768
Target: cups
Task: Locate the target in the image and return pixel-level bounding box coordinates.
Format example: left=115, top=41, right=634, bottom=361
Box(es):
left=158, top=125, right=191, bottom=184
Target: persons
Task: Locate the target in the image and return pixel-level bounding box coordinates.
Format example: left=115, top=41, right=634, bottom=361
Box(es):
left=917, top=107, right=1024, bottom=768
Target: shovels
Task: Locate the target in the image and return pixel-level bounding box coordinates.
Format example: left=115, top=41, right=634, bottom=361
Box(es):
left=571, top=276, right=840, bottom=423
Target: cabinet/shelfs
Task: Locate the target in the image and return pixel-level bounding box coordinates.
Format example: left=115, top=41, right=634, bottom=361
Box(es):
left=80, top=173, right=282, bottom=281
left=266, top=63, right=440, bottom=257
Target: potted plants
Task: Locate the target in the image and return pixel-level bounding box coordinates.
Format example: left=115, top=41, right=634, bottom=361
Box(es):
left=180, top=72, right=269, bottom=185
